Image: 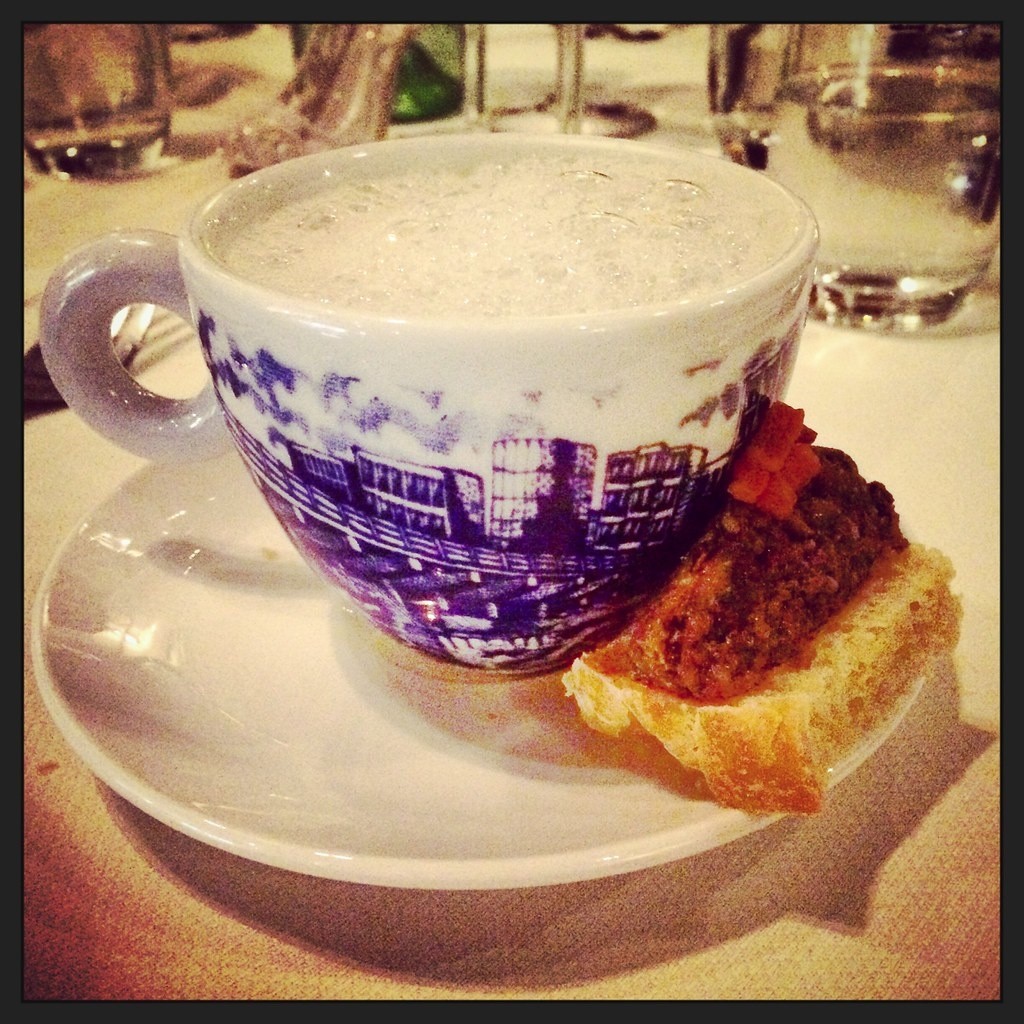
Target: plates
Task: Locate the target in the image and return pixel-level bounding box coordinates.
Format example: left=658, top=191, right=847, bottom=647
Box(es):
left=30, top=443, right=934, bottom=887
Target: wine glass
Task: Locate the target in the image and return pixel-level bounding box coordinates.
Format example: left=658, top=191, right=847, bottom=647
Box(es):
left=403, top=11, right=656, bottom=139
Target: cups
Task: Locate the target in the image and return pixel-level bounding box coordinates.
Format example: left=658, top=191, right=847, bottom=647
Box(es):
left=289, top=22, right=466, bottom=123
left=778, top=68, right=1002, bottom=329
left=39, top=130, right=819, bottom=682
left=22, top=22, right=172, bottom=176
left=706, top=24, right=889, bottom=168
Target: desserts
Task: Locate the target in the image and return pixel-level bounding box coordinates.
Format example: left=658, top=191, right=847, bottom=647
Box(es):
left=559, top=400, right=963, bottom=813
